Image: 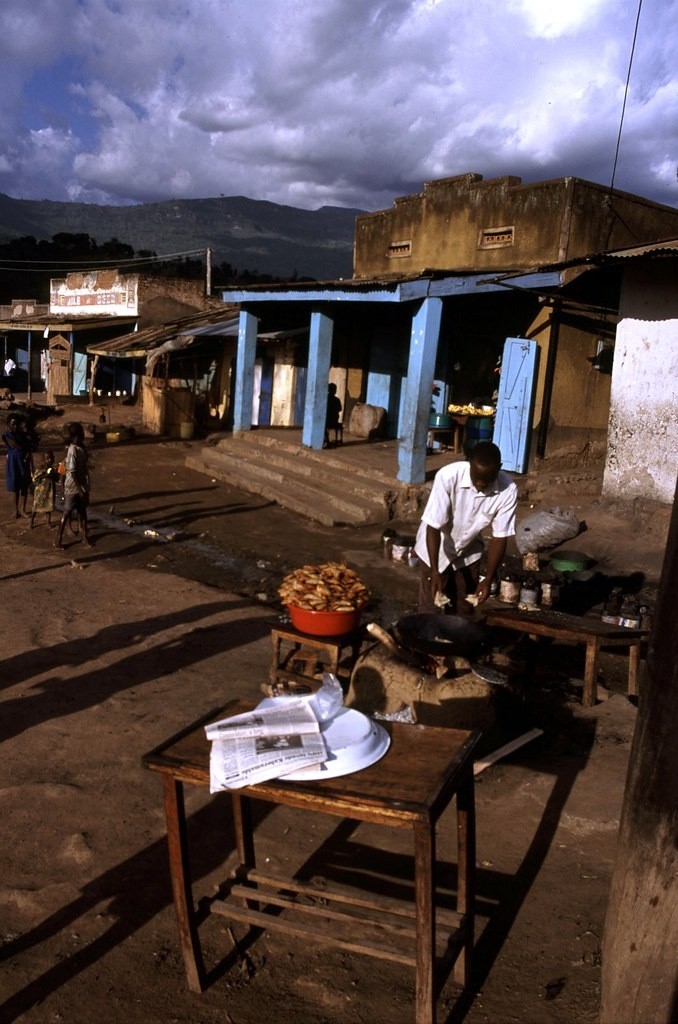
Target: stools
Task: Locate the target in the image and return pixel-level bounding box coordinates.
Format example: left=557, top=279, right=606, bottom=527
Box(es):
left=428, top=430, right=449, bottom=452
left=269, top=625, right=362, bottom=694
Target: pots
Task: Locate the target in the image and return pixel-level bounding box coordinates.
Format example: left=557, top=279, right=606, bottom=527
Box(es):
left=395, top=612, right=482, bottom=656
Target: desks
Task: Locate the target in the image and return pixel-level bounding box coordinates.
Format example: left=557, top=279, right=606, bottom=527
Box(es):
left=448, top=412, right=495, bottom=454
left=481, top=607, right=649, bottom=707
left=139, top=697, right=482, bottom=1024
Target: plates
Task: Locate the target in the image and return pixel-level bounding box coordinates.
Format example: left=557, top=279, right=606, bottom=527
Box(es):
left=278, top=707, right=391, bottom=781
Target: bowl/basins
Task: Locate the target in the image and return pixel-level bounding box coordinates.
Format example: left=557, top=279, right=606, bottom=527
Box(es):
left=287, top=604, right=363, bottom=635
left=429, top=414, right=450, bottom=429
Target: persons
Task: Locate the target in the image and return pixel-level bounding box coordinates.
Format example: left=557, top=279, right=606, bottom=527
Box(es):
left=3, top=354, right=17, bottom=393
left=2, top=412, right=93, bottom=550
left=414, top=442, right=518, bottom=634
left=324, top=383, right=342, bottom=449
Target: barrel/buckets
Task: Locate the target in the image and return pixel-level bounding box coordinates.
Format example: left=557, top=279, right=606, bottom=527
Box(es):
left=466, top=416, right=491, bottom=447
left=180, top=422, right=194, bottom=439
